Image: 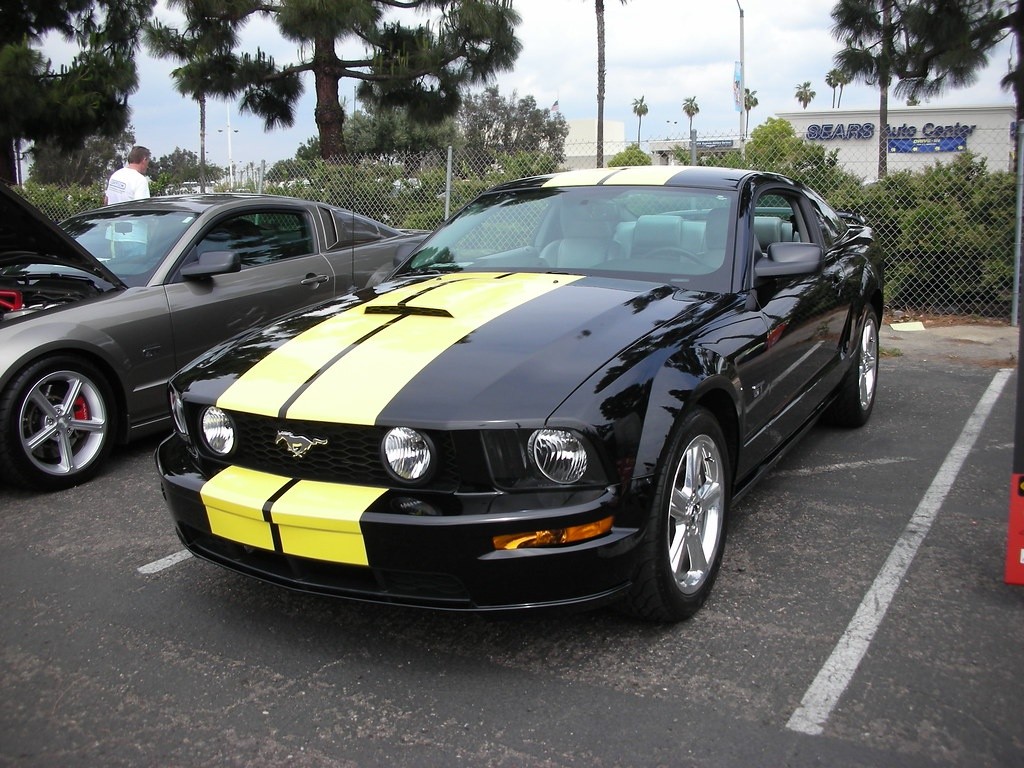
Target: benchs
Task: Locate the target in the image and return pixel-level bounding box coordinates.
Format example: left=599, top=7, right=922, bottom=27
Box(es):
left=238, top=228, right=307, bottom=267
left=614, top=214, right=800, bottom=261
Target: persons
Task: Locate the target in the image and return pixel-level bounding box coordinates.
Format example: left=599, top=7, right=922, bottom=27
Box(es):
left=104, top=145, right=151, bottom=258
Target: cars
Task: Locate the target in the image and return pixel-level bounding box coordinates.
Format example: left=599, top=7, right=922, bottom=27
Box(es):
left=0, top=182, right=434, bottom=499
left=155, top=166, right=884, bottom=630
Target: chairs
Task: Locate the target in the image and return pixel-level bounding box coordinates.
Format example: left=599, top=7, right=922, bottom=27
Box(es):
left=145, top=222, right=185, bottom=261
left=686, top=206, right=728, bottom=268
left=197, top=228, right=251, bottom=268
left=539, top=200, right=625, bottom=268
